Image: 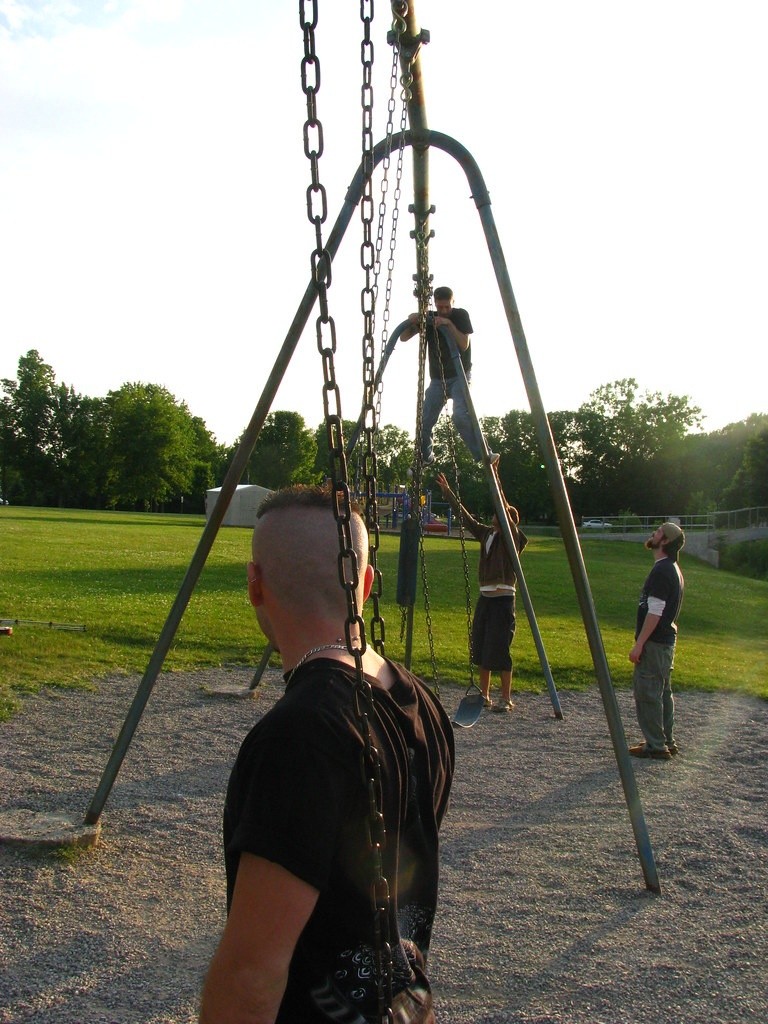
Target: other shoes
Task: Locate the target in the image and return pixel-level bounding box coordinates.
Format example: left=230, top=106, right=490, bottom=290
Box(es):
left=483, top=697, right=492, bottom=707
left=638, top=742, right=678, bottom=755
left=407, top=453, right=436, bottom=476
left=477, top=451, right=500, bottom=469
left=630, top=743, right=671, bottom=759
left=492, top=698, right=514, bottom=711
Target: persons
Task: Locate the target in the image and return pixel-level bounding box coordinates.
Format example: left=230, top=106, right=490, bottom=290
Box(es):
left=625, top=522, right=685, bottom=759
left=435, top=460, right=529, bottom=713
left=195, top=482, right=455, bottom=1024
left=400, top=286, right=500, bottom=466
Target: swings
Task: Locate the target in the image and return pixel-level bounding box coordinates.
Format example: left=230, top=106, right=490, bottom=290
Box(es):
left=408, top=204, right=486, bottom=728
left=299, top=0, right=430, bottom=1024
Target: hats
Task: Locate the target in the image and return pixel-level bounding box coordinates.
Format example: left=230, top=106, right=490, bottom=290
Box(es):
left=661, top=522, right=686, bottom=562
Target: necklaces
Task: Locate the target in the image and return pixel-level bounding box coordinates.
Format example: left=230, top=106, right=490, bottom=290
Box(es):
left=283, top=644, right=371, bottom=689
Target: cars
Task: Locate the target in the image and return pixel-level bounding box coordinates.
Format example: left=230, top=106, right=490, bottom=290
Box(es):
left=582, top=520, right=612, bottom=529
left=421, top=513, right=448, bottom=532
